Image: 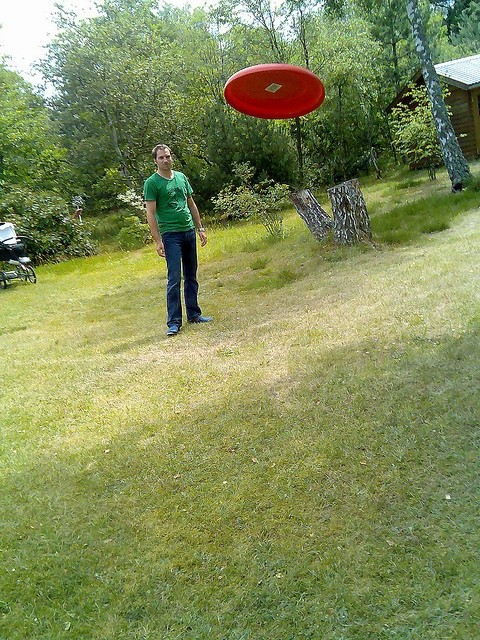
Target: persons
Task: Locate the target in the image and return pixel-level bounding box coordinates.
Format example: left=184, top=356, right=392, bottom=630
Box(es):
left=143, top=144, right=212, bottom=335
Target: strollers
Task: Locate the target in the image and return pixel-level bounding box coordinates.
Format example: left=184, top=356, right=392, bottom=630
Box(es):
left=0, top=222, right=37, bottom=288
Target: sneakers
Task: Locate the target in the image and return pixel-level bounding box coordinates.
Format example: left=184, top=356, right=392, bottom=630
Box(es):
left=167, top=325, right=180, bottom=335
left=188, top=316, right=210, bottom=322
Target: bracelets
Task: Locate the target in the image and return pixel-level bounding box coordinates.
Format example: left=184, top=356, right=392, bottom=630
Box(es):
left=198, top=227, right=205, bottom=232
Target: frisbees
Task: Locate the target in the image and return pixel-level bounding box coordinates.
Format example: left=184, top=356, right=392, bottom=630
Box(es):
left=223, top=63, right=326, bottom=119
